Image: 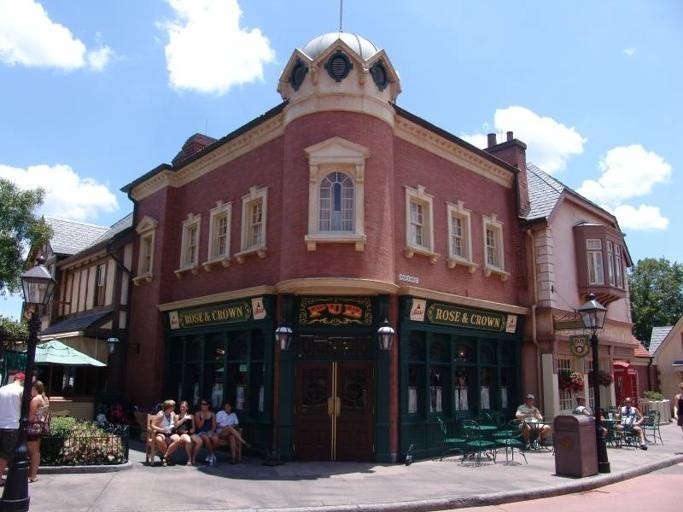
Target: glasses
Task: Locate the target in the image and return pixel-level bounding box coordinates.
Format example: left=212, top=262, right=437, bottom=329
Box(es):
left=200, top=402, right=208, bottom=405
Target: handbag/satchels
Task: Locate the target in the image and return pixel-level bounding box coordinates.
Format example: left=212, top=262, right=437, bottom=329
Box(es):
left=27, top=421, right=45, bottom=435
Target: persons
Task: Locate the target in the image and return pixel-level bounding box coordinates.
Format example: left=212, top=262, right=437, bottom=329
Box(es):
left=43, top=376, right=142, bottom=441
left=673, top=383, right=682, bottom=433
left=149, top=399, right=193, bottom=466
left=599, top=397, right=648, bottom=450
left=194, top=398, right=218, bottom=463
left=573, top=396, right=591, bottom=417
left=214, top=401, right=250, bottom=464
left=150, top=403, right=163, bottom=415
left=0, top=371, right=27, bottom=488
left=175, top=398, right=203, bottom=466
left=514, top=393, right=551, bottom=449
left=25, top=378, right=49, bottom=482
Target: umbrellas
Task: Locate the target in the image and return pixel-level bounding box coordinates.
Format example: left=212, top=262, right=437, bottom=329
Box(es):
left=22, top=341, right=107, bottom=391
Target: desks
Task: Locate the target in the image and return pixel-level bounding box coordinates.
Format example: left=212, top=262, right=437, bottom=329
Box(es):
left=638, top=398, right=671, bottom=424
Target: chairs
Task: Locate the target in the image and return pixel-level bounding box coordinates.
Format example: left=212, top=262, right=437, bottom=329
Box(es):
left=434, top=403, right=664, bottom=468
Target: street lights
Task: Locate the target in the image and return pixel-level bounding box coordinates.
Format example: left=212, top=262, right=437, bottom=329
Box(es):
left=577, top=292, right=612, bottom=475
left=0, top=256, right=60, bottom=510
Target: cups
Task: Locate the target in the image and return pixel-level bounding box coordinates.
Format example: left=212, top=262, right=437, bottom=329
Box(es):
left=608, top=412, right=620, bottom=420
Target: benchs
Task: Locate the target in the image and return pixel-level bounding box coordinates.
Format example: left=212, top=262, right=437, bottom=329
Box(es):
left=143, top=413, right=245, bottom=466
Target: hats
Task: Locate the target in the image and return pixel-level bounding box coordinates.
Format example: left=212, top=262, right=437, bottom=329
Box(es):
left=14, top=372, right=25, bottom=380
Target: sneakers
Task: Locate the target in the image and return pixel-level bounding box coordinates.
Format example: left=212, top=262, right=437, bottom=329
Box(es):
left=639, top=445, right=647, bottom=450
left=205, top=455, right=210, bottom=463
left=525, top=440, right=541, bottom=450
left=208, top=456, right=216, bottom=465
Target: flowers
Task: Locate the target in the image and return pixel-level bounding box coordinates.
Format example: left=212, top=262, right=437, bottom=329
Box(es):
left=556, top=369, right=584, bottom=395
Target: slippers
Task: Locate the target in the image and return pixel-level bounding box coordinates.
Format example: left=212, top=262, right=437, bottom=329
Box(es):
left=27, top=476, right=38, bottom=483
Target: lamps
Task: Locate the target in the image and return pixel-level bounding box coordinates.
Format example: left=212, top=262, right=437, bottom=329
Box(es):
left=274, top=321, right=293, bottom=352
left=377, top=315, right=396, bottom=353
left=104, top=335, right=121, bottom=356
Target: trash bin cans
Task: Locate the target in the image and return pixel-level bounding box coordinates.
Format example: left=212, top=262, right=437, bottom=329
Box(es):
left=553, top=414, right=598, bottom=478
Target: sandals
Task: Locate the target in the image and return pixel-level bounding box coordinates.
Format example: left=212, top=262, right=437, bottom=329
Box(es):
left=160, top=456, right=175, bottom=467
